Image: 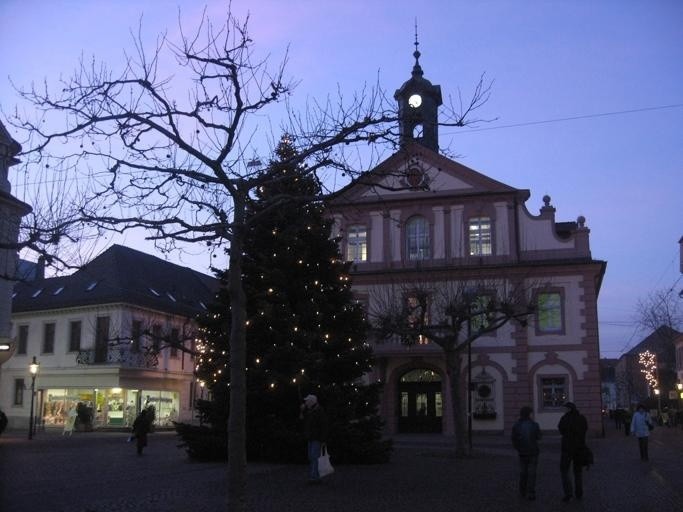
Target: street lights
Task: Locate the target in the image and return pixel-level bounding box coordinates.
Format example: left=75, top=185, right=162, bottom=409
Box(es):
left=28, top=356, right=41, bottom=441
left=462, top=277, right=477, bottom=451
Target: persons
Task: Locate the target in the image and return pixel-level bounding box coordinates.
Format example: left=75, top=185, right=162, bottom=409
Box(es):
left=557, top=402, right=593, bottom=501
left=614, top=402, right=622, bottom=431
left=127, top=409, right=153, bottom=454
left=624, top=415, right=631, bottom=435
left=631, top=402, right=654, bottom=462
left=511, top=405, right=543, bottom=499
left=298, top=394, right=334, bottom=483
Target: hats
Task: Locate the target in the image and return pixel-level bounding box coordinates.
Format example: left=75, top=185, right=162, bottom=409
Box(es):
left=304, top=395, right=317, bottom=403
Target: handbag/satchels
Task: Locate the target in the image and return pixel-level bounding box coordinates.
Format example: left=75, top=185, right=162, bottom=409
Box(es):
left=645, top=420, right=654, bottom=431
left=578, top=444, right=594, bottom=467
left=318, top=455, right=334, bottom=478
